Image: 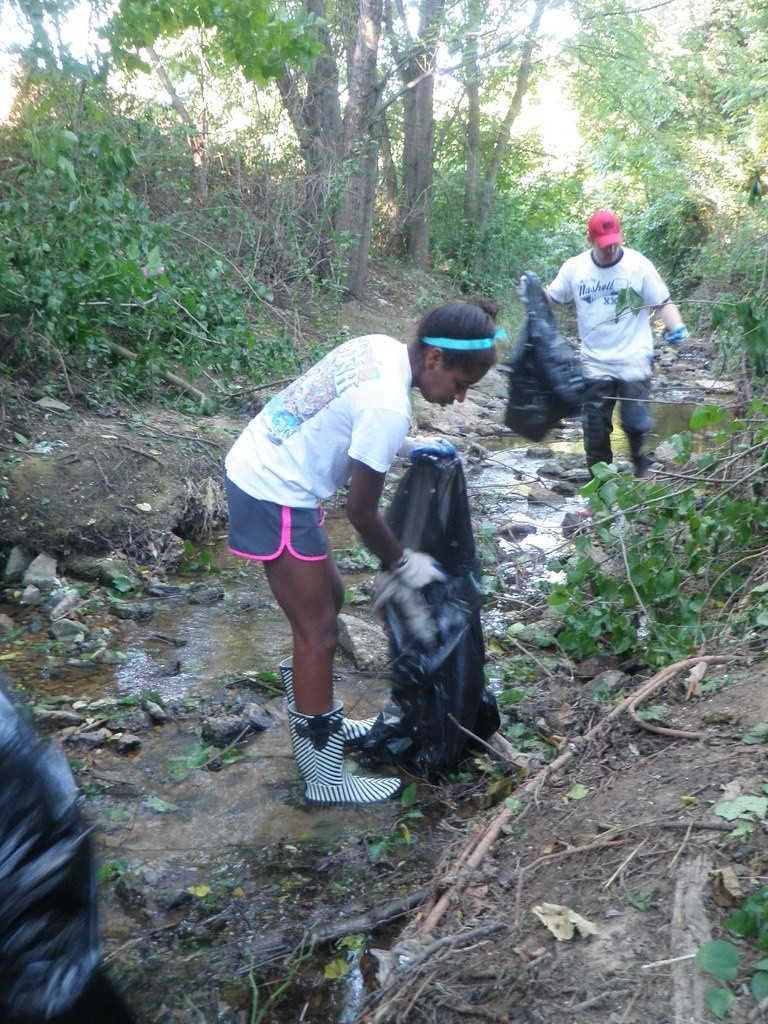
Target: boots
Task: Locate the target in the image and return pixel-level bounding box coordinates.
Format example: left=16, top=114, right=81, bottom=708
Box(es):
left=287, top=700, right=404, bottom=806
left=279, top=654, right=380, bottom=746
left=617, top=379, right=663, bottom=466
left=582, top=378, right=618, bottom=479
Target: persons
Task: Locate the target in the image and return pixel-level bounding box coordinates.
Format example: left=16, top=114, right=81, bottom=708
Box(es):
left=223, top=293, right=503, bottom=806
left=518, top=208, right=689, bottom=490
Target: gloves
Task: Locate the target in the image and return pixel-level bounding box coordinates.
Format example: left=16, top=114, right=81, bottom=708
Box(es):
left=392, top=547, right=447, bottom=589
left=665, top=323, right=687, bottom=348
left=396, top=436, right=456, bottom=462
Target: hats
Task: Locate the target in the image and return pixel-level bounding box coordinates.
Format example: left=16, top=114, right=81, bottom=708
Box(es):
left=589, top=211, right=622, bottom=248
